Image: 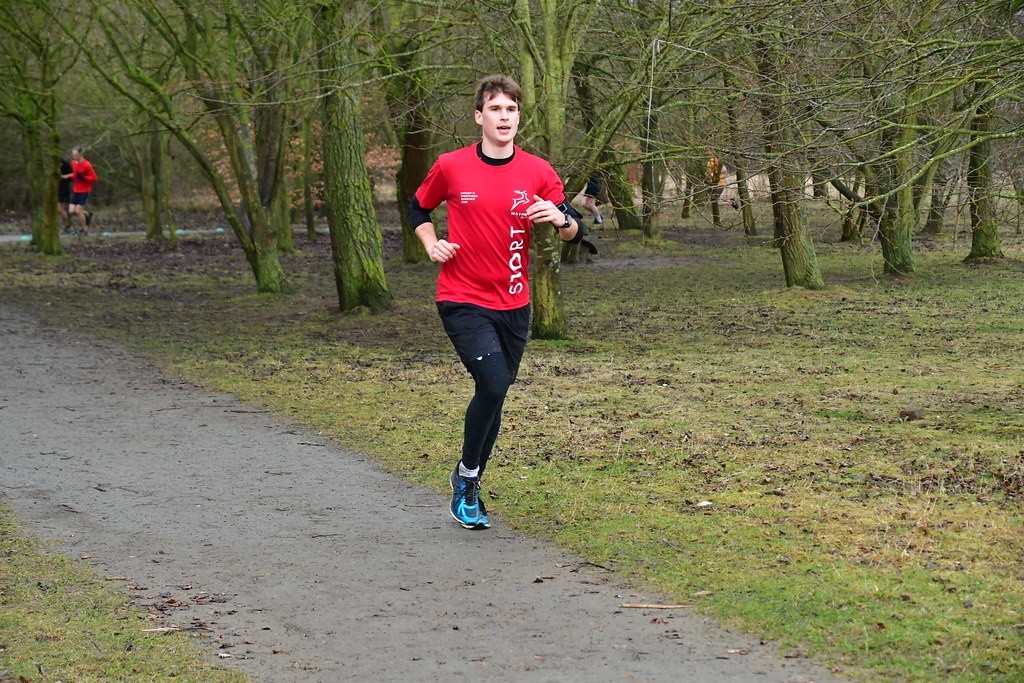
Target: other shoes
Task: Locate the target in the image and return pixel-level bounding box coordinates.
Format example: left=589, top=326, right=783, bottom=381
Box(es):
left=73, top=229, right=88, bottom=235
left=86, top=212, right=93, bottom=226
left=730, top=198, right=739, bottom=210
left=593, top=214, right=604, bottom=224
left=61, top=227, right=71, bottom=234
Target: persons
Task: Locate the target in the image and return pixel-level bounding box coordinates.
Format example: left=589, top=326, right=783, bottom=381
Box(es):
left=59, top=155, right=73, bottom=234
left=406, top=76, right=582, bottom=531
left=582, top=167, right=606, bottom=224
left=702, top=148, right=740, bottom=214
left=69, top=146, right=97, bottom=234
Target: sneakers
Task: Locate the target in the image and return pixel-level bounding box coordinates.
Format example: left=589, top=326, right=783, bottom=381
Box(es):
left=449, top=459, right=478, bottom=526
left=459, top=495, right=490, bottom=529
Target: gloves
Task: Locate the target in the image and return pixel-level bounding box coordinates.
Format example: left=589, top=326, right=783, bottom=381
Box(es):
left=76, top=172, right=84, bottom=180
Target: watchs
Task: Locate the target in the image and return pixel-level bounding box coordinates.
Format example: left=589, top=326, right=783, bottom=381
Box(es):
left=555, top=213, right=571, bottom=229
